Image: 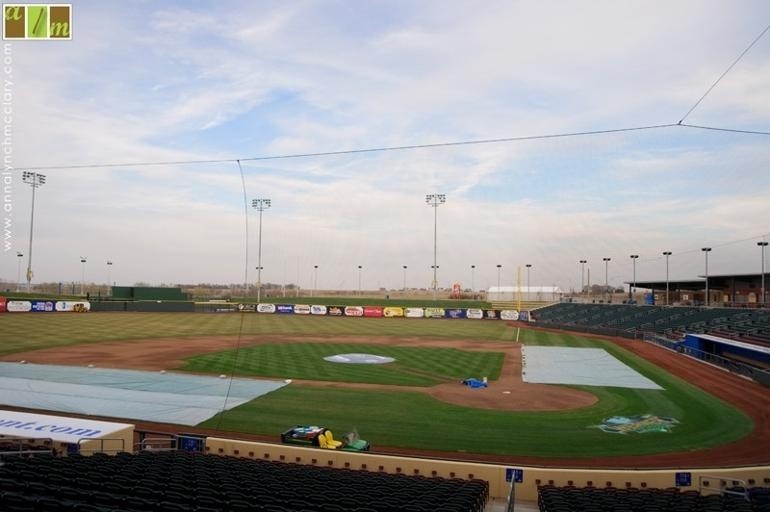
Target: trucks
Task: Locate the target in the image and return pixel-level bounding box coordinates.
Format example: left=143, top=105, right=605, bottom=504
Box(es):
left=281, top=424, right=369, bottom=453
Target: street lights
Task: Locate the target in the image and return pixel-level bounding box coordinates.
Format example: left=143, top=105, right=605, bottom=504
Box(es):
left=17, top=254, right=112, bottom=296
left=579, top=241, right=768, bottom=306
left=251, top=198, right=271, bottom=302
left=22, top=169, right=46, bottom=294
left=256, top=264, right=531, bottom=299
left=425, top=192, right=446, bottom=300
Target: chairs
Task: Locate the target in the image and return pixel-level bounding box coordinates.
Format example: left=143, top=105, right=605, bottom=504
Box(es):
left=0, top=441, right=770, bottom=512
left=318, top=434, right=336, bottom=449
left=325, top=431, right=342, bottom=447
